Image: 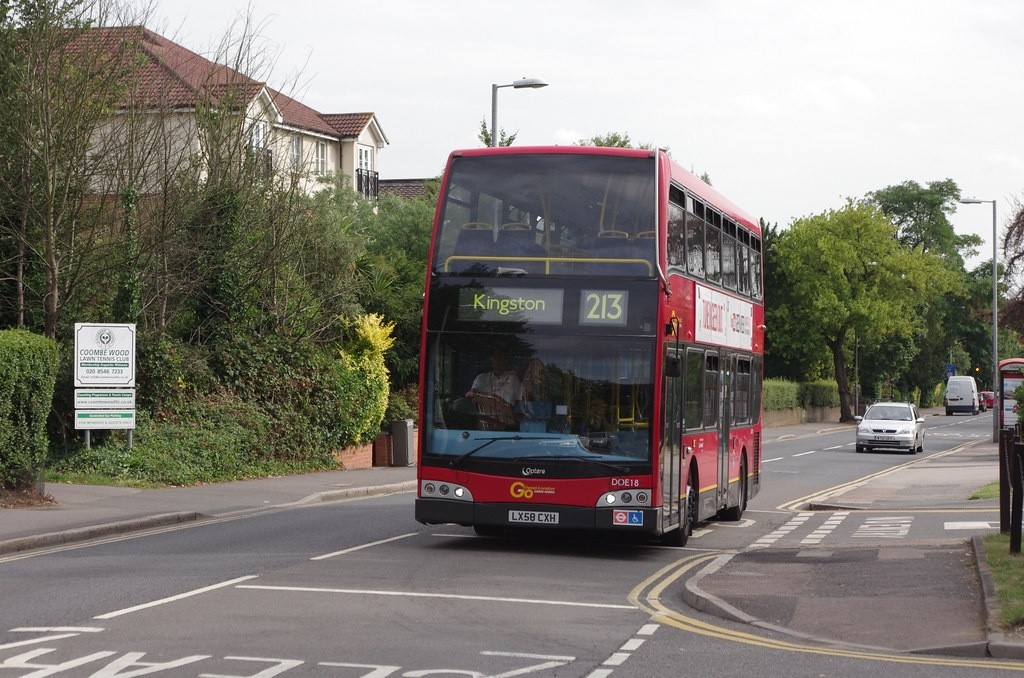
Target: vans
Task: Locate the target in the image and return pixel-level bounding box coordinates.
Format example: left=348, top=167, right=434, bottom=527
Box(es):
left=946, top=376, right=980, bottom=415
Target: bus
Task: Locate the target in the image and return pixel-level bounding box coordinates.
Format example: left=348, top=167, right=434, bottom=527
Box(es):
left=415, top=145, right=765, bottom=544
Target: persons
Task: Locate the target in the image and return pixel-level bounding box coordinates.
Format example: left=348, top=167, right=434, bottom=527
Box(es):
left=465, top=345, right=529, bottom=430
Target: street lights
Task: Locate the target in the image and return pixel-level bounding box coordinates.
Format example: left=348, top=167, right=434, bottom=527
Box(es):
left=959, top=198, right=999, bottom=442
left=491, top=78, right=548, bottom=147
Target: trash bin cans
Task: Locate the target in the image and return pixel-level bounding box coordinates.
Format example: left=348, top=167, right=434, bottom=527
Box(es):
left=392, top=418, right=415, bottom=467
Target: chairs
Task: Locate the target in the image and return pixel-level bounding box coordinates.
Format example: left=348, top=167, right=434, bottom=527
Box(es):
left=496, top=223, right=535, bottom=274
left=452, top=221, right=496, bottom=273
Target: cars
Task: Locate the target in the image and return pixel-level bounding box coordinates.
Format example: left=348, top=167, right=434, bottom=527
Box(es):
left=983, top=391, right=1000, bottom=408
left=977, top=392, right=986, bottom=411
left=855, top=402, right=926, bottom=454
